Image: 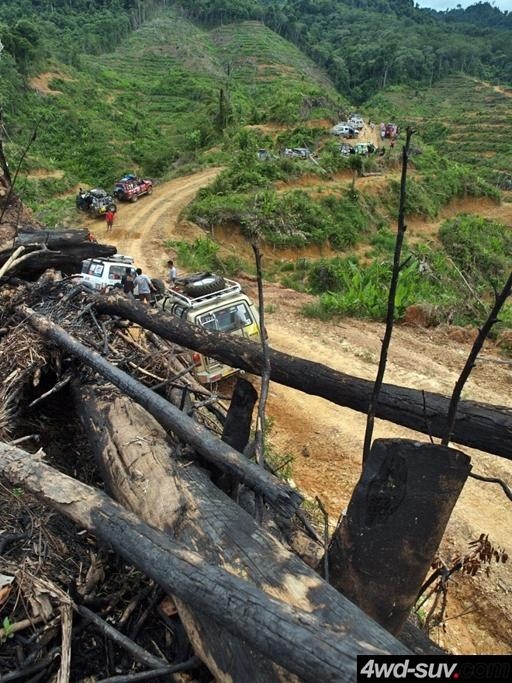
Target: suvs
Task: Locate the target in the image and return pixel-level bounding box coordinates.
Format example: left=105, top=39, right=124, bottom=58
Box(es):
left=72, top=254, right=141, bottom=295
left=113, top=175, right=153, bottom=201
left=79, top=188, right=118, bottom=218
left=144, top=270, right=267, bottom=386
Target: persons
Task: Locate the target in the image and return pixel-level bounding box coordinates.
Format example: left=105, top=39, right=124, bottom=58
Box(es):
left=105, top=208, right=113, bottom=231
left=165, top=260, right=177, bottom=289
left=132, top=268, right=158, bottom=303
left=87, top=232, right=97, bottom=244
left=79, top=188, right=85, bottom=209
left=348, top=119, right=401, bottom=157
left=121, top=268, right=136, bottom=326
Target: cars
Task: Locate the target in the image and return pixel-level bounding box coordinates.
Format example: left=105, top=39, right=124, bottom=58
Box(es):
left=255, top=115, right=400, bottom=162
